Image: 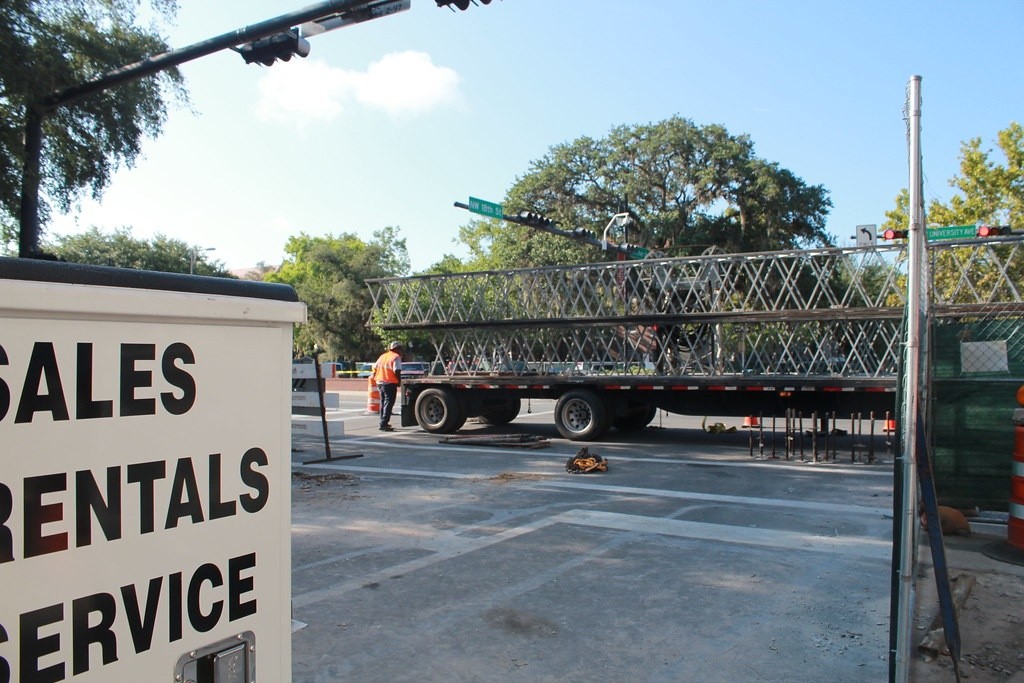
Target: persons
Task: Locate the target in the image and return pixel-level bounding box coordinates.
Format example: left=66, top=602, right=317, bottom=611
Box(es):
left=371, top=341, right=402, bottom=431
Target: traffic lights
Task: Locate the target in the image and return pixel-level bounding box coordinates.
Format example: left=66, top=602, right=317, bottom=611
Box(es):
left=884, top=229, right=909, bottom=239
left=245, top=28, right=314, bottom=68
left=519, top=210, right=549, bottom=227
left=977, top=225, right=1012, bottom=237
left=618, top=241, right=638, bottom=253
left=570, top=226, right=596, bottom=241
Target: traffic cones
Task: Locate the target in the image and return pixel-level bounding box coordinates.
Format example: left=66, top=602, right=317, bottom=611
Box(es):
left=882, top=420, right=895, bottom=432
left=742, top=415, right=760, bottom=428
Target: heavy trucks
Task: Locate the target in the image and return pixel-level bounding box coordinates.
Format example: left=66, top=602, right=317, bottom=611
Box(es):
left=364, top=235, right=1023, bottom=443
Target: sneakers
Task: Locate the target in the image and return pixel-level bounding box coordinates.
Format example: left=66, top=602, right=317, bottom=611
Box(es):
left=379, top=424, right=393, bottom=431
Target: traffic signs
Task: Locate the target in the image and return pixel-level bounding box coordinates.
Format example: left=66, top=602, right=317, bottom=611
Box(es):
left=468, top=196, right=504, bottom=220
left=924, top=224, right=976, bottom=240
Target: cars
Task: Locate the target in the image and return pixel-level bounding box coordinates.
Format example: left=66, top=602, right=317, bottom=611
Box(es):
left=322, top=360, right=692, bottom=379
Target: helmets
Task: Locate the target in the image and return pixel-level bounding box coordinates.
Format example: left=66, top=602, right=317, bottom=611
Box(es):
left=390, top=340, right=402, bottom=350
